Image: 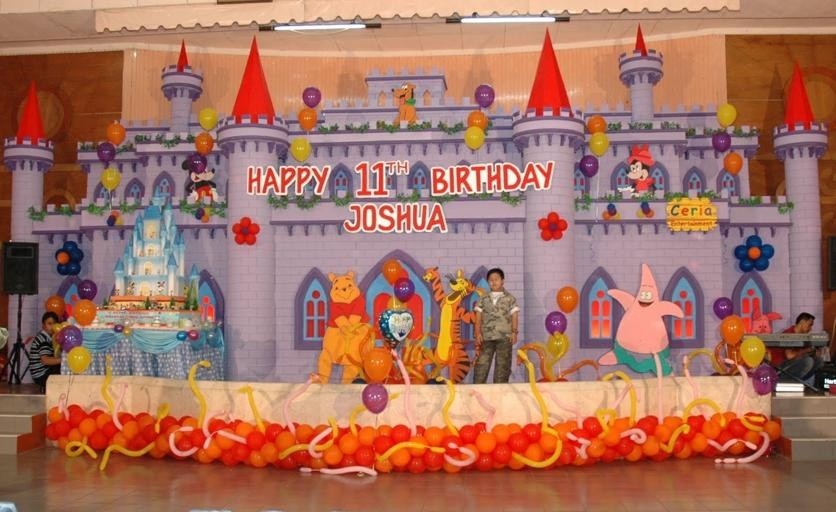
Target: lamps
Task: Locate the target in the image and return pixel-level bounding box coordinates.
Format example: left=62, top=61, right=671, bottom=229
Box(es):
left=444, top=14, right=571, bottom=25
left=258, top=20, right=382, bottom=32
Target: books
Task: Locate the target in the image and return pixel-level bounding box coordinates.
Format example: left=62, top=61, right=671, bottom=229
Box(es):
left=188, top=329, right=199, bottom=340
left=176, top=330, right=187, bottom=340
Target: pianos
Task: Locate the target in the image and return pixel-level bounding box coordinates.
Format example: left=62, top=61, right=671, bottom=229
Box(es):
left=741, top=332, right=829, bottom=348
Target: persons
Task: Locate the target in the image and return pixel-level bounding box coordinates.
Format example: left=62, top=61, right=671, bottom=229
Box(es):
left=767, top=312, right=824, bottom=391
left=28, top=311, right=61, bottom=388
left=471, top=268, right=519, bottom=383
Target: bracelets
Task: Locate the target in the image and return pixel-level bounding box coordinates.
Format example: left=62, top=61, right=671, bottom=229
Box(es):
left=513, top=328, right=519, bottom=334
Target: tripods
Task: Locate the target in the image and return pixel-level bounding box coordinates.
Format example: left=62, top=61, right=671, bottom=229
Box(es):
left=0, top=294, right=31, bottom=385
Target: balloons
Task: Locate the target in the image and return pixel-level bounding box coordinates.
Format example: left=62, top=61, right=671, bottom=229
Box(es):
left=393, top=277, right=415, bottom=304
left=98, top=124, right=126, bottom=191
left=107, top=210, right=123, bottom=228
left=44, top=279, right=97, bottom=372
left=732, top=234, right=775, bottom=271
left=465, top=84, right=495, bottom=149
left=123, top=326, right=132, bottom=336
left=382, top=257, right=401, bottom=282
left=114, top=324, right=124, bottom=333
left=721, top=313, right=744, bottom=346
left=54, top=240, right=84, bottom=276
left=579, top=116, right=609, bottom=176
left=739, top=335, right=768, bottom=368
left=291, top=87, right=321, bottom=161
left=40, top=411, right=783, bottom=474
left=545, top=311, right=568, bottom=335
left=379, top=307, right=416, bottom=345
left=601, top=201, right=653, bottom=220
left=194, top=207, right=210, bottom=223
left=712, top=104, right=742, bottom=176
left=753, top=364, right=779, bottom=396
left=713, top=297, right=734, bottom=319
left=206, top=331, right=215, bottom=340
left=537, top=212, right=567, bottom=241
left=548, top=333, right=571, bottom=358
left=362, top=382, right=389, bottom=414
left=557, top=286, right=581, bottom=313
left=364, top=346, right=395, bottom=380
left=232, top=217, right=258, bottom=246
left=188, top=108, right=218, bottom=173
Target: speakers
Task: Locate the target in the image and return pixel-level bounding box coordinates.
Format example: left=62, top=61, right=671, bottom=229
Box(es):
left=0, top=240, right=41, bottom=294
left=826, top=234, right=836, bottom=291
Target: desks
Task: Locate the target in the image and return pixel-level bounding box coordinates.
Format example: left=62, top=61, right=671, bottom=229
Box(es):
left=60, top=325, right=226, bottom=383
